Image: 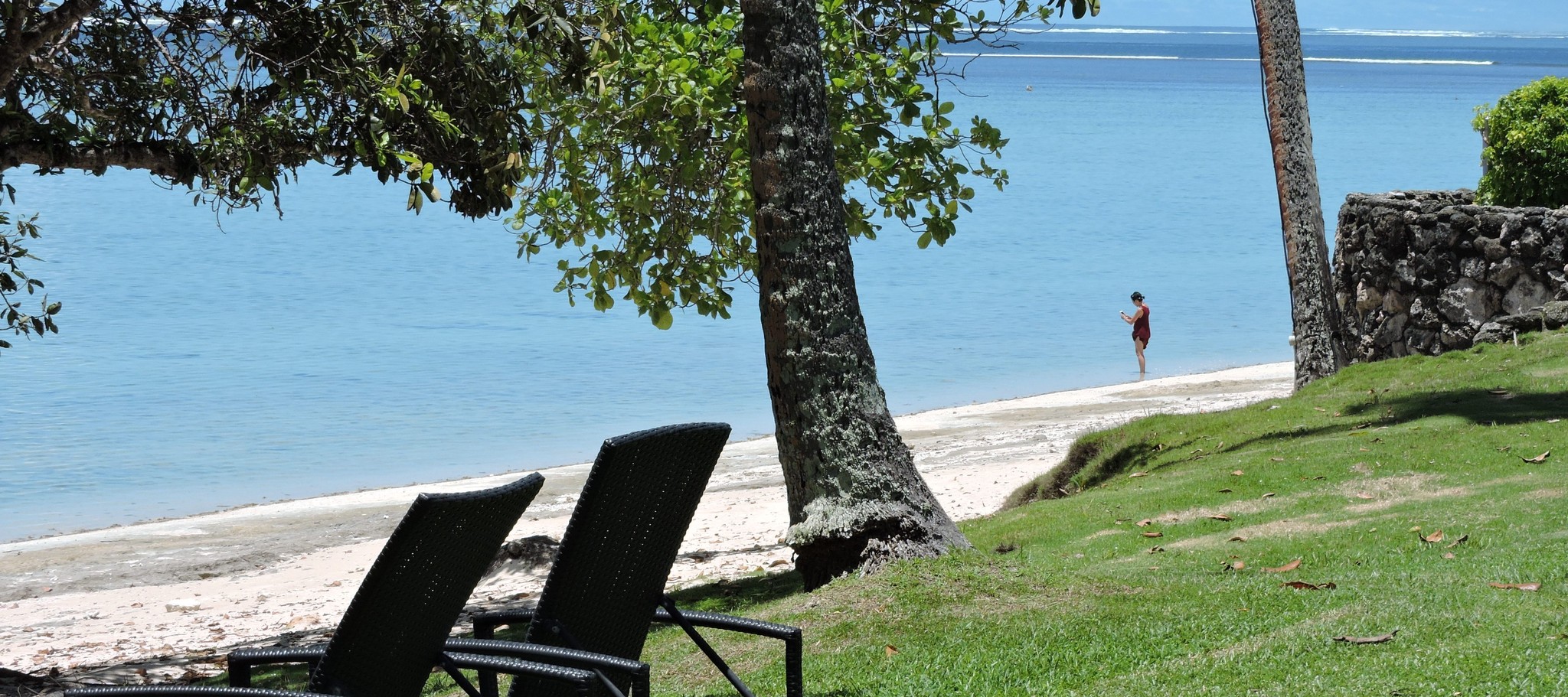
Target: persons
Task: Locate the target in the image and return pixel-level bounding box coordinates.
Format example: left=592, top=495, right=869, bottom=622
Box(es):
left=1120, top=291, right=1151, bottom=382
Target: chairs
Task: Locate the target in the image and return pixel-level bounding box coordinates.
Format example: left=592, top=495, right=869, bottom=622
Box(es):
left=62, top=470, right=544, bottom=697
left=441, top=423, right=804, bottom=696
